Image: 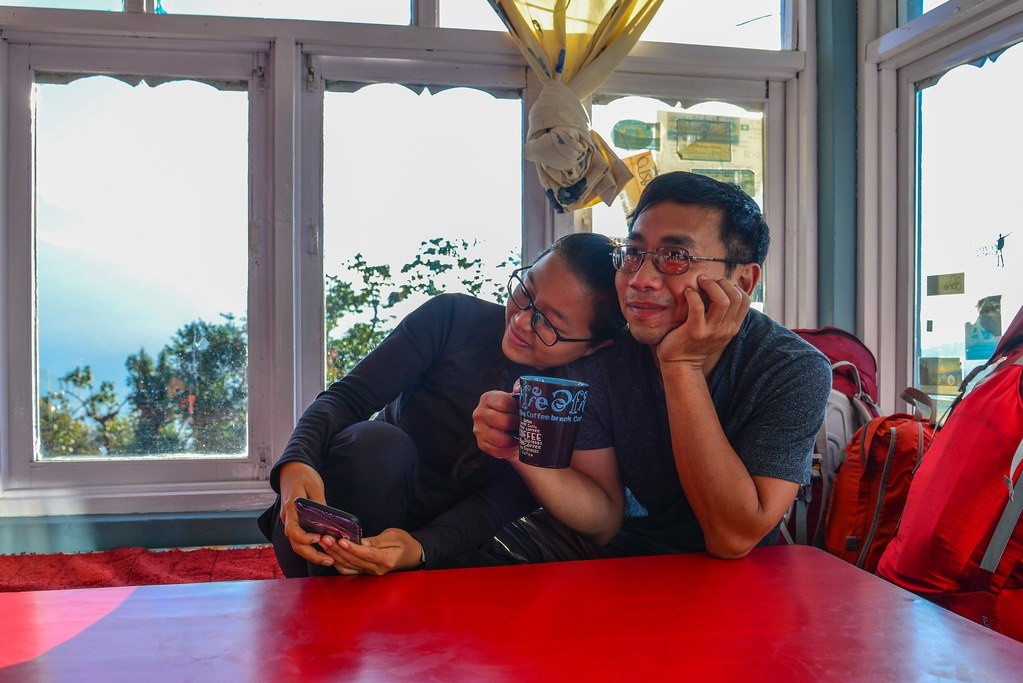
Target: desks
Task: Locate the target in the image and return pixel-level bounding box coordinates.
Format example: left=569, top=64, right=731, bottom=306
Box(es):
left=0, top=543, right=1023, bottom=683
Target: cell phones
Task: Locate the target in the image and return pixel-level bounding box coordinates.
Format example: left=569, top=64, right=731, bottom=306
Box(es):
left=294, top=496, right=362, bottom=557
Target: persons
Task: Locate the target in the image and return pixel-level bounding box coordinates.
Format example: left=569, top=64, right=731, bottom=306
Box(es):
left=256, top=233, right=624, bottom=578
left=473, top=169, right=832, bottom=560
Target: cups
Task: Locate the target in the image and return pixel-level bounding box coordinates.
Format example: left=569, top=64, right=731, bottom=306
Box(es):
left=510, top=375, right=590, bottom=469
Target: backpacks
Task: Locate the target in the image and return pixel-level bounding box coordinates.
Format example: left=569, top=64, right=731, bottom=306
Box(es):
left=777, top=357, right=945, bottom=577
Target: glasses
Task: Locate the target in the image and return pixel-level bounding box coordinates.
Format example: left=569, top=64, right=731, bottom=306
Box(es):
left=507, top=265, right=594, bottom=346
left=609, top=245, right=745, bottom=276
